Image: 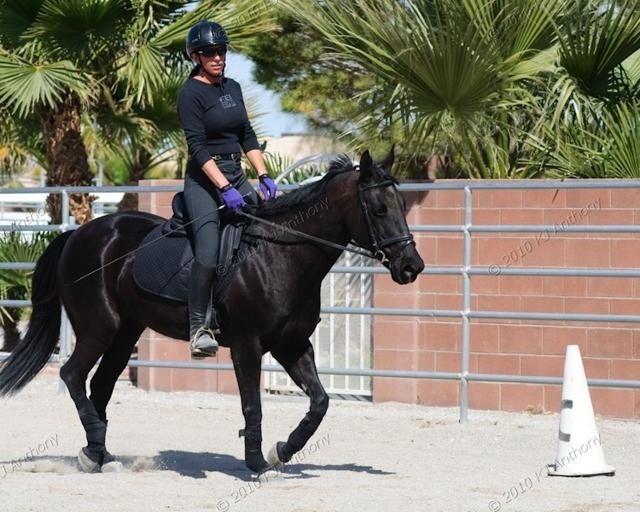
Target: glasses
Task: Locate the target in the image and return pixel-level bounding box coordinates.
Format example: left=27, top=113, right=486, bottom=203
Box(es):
left=199, top=48, right=227, bottom=57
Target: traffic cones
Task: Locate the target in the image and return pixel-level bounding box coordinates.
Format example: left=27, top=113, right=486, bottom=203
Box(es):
left=546, top=343, right=615, bottom=477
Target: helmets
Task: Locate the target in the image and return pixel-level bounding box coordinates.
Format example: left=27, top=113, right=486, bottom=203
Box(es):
left=186, top=22, right=229, bottom=55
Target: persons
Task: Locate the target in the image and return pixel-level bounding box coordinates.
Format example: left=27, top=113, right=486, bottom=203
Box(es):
left=177, top=21, right=279, bottom=358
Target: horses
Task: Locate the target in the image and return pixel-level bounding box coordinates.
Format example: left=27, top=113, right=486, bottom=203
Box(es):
left=0, top=141, right=426, bottom=480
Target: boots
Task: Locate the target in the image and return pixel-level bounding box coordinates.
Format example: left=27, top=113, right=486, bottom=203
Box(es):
left=188, top=260, right=220, bottom=360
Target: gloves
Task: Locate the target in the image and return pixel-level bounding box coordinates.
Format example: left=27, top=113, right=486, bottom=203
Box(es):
left=221, top=184, right=245, bottom=210
left=258, top=173, right=277, bottom=200
